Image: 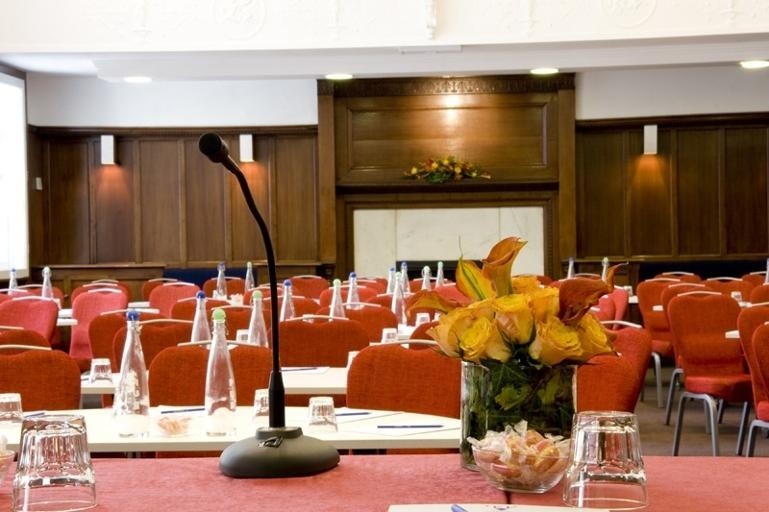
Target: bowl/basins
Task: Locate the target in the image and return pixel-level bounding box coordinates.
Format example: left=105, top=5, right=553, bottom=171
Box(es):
left=469, top=439, right=568, bottom=494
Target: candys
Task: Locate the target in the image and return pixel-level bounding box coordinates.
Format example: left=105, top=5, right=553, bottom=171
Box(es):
left=466, top=420, right=570, bottom=487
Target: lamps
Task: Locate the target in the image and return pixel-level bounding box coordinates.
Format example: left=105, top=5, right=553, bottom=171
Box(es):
left=100, top=132, right=115, bottom=166
left=238, top=133, right=255, bottom=163
left=641, top=121, right=659, bottom=155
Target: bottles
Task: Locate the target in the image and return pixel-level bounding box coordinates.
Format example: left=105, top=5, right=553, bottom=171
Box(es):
left=421, top=266, right=432, bottom=291
left=400, top=261, right=411, bottom=298
left=392, top=272, right=407, bottom=336
left=245, top=261, right=255, bottom=292
left=42, top=268, right=54, bottom=300
left=113, top=311, right=150, bottom=435
left=248, top=291, right=269, bottom=349
left=215, top=263, right=228, bottom=300
left=204, top=308, right=237, bottom=435
left=347, top=272, right=361, bottom=311
left=601, top=257, right=609, bottom=282
left=279, top=279, right=295, bottom=322
left=8, top=269, right=17, bottom=295
left=328, top=279, right=345, bottom=323
left=387, top=266, right=395, bottom=294
left=435, top=261, right=445, bottom=289
left=566, top=257, right=576, bottom=279
left=191, top=290, right=210, bottom=343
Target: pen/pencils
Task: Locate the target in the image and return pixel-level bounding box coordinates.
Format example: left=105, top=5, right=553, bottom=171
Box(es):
left=377, top=425, right=444, bottom=428
left=335, top=412, right=372, bottom=416
left=80, top=378, right=90, bottom=381
left=450, top=504, right=468, bottom=512
left=24, top=412, right=45, bottom=418
left=279, top=367, right=317, bottom=372
left=161, top=408, right=205, bottom=414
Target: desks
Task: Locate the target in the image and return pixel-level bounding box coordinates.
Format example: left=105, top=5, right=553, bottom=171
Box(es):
left=0, top=452, right=769, bottom=511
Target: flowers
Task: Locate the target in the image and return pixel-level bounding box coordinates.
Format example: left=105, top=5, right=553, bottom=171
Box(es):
left=395, top=154, right=492, bottom=184
left=399, top=236, right=628, bottom=460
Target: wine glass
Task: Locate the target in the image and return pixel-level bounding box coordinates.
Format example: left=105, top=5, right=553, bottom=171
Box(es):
left=0, top=395, right=25, bottom=495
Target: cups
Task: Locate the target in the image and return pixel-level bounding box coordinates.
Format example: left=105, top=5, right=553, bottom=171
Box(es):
left=730, top=291, right=742, bottom=303
left=416, top=313, right=430, bottom=329
left=14, top=415, right=98, bottom=512
left=251, top=388, right=269, bottom=431
left=90, top=357, right=112, bottom=384
left=347, top=350, right=360, bottom=371
left=625, top=285, right=633, bottom=297
left=306, top=396, right=338, bottom=435
left=302, top=314, right=314, bottom=323
left=562, top=410, right=647, bottom=510
left=235, top=328, right=249, bottom=345
left=381, top=327, right=398, bottom=344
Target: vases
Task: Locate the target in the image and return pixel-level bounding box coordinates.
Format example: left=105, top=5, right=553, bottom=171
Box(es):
left=458, top=354, right=580, bottom=478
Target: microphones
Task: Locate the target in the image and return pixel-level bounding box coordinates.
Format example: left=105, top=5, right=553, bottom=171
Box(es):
left=197, top=130, right=340, bottom=478
